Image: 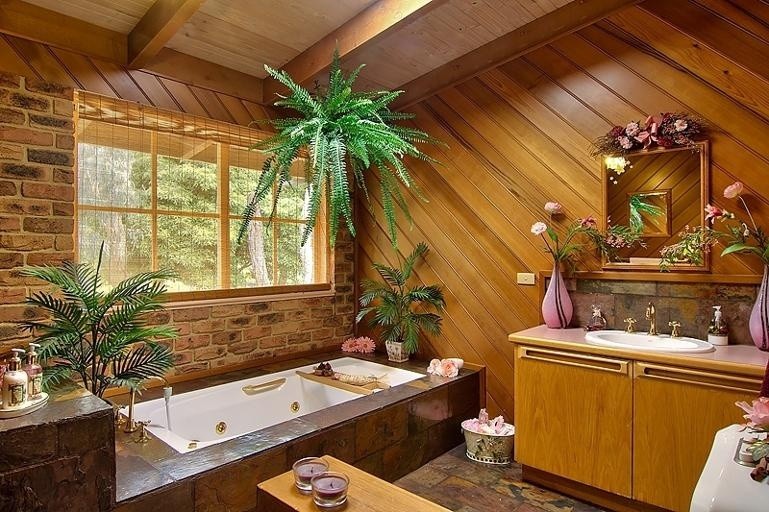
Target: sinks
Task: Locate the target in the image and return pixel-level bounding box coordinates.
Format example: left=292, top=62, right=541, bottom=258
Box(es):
left=585, top=329, right=715, bottom=354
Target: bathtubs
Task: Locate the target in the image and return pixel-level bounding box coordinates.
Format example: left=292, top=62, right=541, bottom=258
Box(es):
left=118, top=355, right=428, bottom=454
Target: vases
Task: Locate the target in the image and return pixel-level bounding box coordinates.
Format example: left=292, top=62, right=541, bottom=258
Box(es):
left=543, top=267, right=574, bottom=328
left=607, top=246, right=633, bottom=264
left=751, top=275, right=763, bottom=349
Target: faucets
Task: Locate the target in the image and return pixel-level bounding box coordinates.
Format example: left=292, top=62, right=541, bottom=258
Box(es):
left=124, top=374, right=173, bottom=432
left=644, top=301, right=657, bottom=336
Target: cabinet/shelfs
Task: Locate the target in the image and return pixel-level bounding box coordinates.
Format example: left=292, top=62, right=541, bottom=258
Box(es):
left=512, top=343, right=634, bottom=509
left=631, top=353, right=765, bottom=512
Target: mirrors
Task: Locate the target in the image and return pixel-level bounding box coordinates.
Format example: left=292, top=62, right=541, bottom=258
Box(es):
left=600, top=142, right=711, bottom=272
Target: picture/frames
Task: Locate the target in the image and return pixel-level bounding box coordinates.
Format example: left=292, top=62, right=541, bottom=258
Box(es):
left=628, top=189, right=672, bottom=239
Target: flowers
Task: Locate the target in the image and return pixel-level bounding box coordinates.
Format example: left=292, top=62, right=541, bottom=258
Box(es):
left=701, top=183, right=764, bottom=272
left=659, top=226, right=704, bottom=271
left=586, top=111, right=710, bottom=158
left=532, top=203, right=610, bottom=267
left=603, top=224, right=647, bottom=249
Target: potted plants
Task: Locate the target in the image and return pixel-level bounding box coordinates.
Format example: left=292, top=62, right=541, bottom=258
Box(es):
left=356, top=242, right=446, bottom=363
